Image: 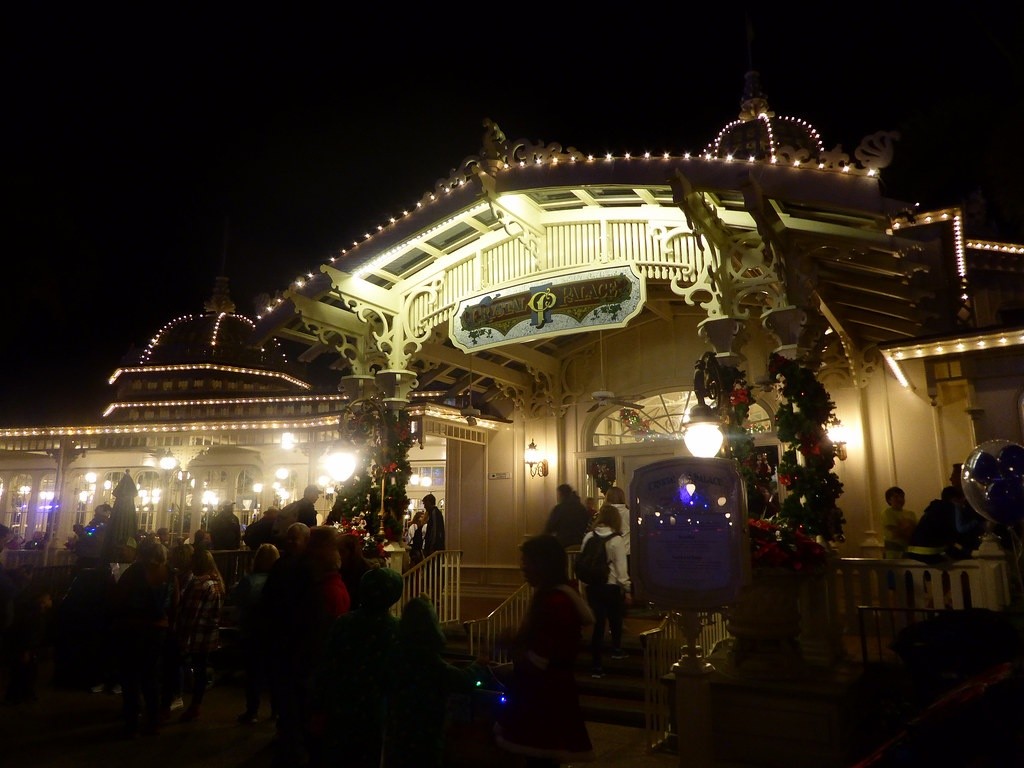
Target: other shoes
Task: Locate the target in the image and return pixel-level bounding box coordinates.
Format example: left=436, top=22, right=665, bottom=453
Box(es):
left=183, top=705, right=201, bottom=721
left=239, top=712, right=258, bottom=723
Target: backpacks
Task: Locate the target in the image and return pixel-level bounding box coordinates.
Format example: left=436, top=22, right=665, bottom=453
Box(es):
left=271, top=499, right=303, bottom=539
left=574, top=530, right=624, bottom=586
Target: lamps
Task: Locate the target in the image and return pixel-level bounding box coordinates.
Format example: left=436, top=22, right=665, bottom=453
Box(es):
left=525, top=438, right=549, bottom=478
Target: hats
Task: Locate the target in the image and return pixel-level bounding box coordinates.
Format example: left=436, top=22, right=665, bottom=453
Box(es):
left=222, top=500, right=236, bottom=510
left=117, top=536, right=137, bottom=549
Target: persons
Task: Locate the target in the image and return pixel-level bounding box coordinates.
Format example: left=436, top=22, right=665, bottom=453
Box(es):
left=1, top=463, right=1024, bottom=768
